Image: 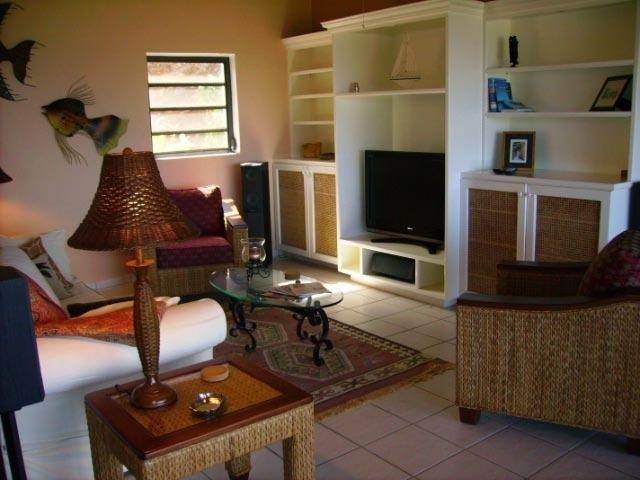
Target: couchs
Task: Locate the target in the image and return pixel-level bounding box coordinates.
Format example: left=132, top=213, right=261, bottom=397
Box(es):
left=2, top=227, right=230, bottom=479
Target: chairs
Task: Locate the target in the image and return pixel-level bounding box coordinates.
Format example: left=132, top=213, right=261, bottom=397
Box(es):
left=453, top=223, right=640, bottom=456
left=143, top=184, right=250, bottom=297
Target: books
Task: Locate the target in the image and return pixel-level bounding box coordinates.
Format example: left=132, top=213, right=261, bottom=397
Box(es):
left=495, top=80, right=512, bottom=111
left=260, top=282, right=331, bottom=300
left=501, top=100, right=533, bottom=112
left=488, top=77, right=507, bottom=112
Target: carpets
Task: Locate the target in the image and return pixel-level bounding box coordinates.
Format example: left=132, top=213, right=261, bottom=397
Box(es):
left=213, top=296, right=457, bottom=424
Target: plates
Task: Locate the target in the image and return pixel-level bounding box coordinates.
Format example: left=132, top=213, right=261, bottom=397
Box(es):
left=493, top=167, right=519, bottom=176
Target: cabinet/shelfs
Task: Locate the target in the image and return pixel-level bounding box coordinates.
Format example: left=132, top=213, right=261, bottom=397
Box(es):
left=457, top=0, right=640, bottom=294
left=321, top=1, right=485, bottom=310
left=272, top=160, right=337, bottom=268
left=281, top=30, right=336, bottom=160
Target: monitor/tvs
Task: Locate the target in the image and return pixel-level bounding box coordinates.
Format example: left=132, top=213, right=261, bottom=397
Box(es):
left=364, top=149, right=445, bottom=254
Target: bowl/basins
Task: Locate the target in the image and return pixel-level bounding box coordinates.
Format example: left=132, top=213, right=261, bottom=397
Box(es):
left=188, top=392, right=227, bottom=415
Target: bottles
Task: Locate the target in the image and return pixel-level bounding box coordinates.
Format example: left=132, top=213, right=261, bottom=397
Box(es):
left=238, top=237, right=267, bottom=265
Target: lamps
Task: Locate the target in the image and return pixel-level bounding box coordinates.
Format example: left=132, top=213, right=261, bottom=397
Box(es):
left=67, top=145, right=201, bottom=410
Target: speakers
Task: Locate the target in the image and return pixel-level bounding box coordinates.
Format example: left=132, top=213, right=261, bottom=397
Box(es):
left=628, top=182, right=640, bottom=231
left=240, top=161, right=272, bottom=265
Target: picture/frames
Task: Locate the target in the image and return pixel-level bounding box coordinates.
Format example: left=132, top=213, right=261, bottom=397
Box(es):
left=494, top=129, right=538, bottom=177
left=590, top=73, right=632, bottom=112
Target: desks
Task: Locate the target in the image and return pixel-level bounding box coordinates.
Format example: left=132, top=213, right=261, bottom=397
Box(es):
left=84, top=352, right=317, bottom=480
